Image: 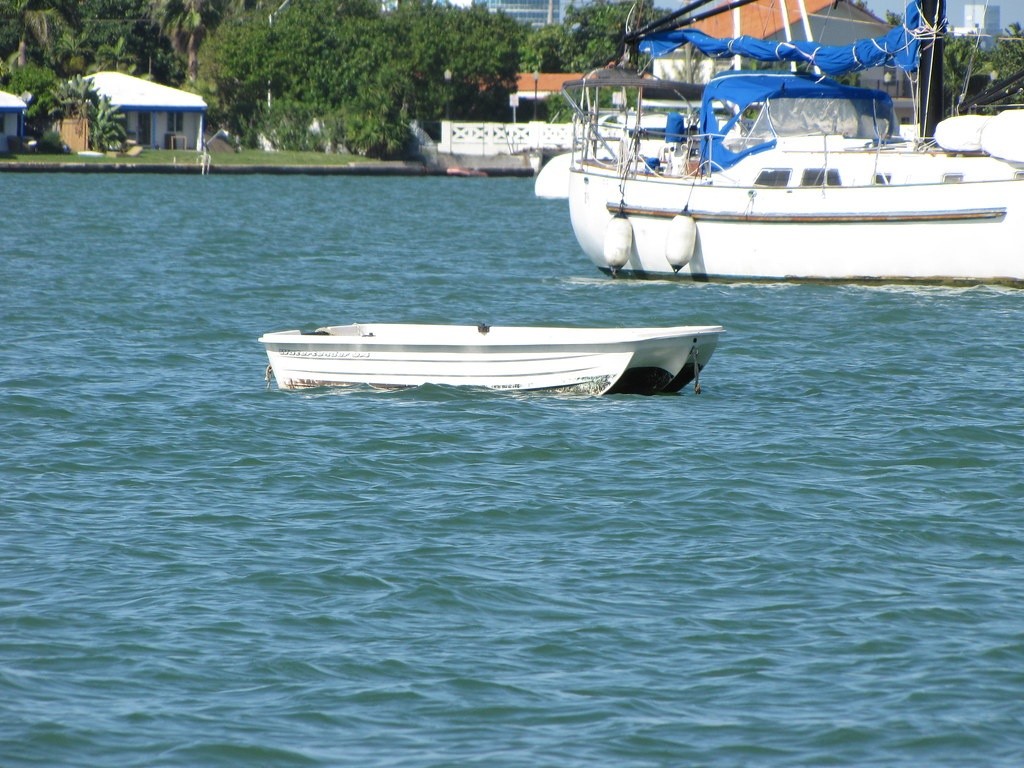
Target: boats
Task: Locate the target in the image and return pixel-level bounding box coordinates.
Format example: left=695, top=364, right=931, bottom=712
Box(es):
left=446, top=169, right=486, bottom=177
left=258, top=321, right=724, bottom=397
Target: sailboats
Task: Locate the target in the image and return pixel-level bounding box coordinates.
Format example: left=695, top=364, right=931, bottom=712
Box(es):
left=535, top=1, right=1024, bottom=286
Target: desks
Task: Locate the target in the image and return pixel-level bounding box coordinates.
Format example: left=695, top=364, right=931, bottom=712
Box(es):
left=168, top=135, right=187, bottom=150
